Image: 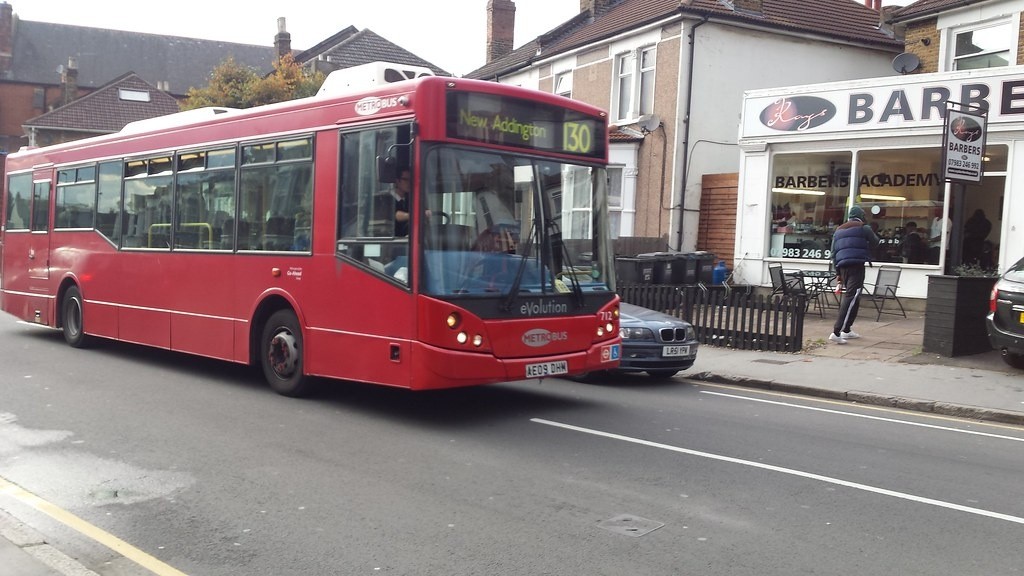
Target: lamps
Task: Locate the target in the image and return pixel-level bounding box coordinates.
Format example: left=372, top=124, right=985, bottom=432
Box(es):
left=636, top=113, right=663, bottom=134
left=920, top=38, right=930, bottom=45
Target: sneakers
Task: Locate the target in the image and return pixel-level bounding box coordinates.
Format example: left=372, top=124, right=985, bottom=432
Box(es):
left=828, top=332, right=847, bottom=343
left=840, top=329, right=860, bottom=339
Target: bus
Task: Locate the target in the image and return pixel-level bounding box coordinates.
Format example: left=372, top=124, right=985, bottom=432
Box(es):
left=0, top=59, right=621, bottom=393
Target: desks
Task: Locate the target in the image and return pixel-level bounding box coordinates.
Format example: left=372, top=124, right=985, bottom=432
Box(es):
left=785, top=274, right=837, bottom=319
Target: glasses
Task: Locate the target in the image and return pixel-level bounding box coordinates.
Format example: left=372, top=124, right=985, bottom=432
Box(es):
left=398, top=178, right=410, bottom=181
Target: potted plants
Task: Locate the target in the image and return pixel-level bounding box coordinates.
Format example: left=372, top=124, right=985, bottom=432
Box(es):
left=921, top=261, right=1002, bottom=358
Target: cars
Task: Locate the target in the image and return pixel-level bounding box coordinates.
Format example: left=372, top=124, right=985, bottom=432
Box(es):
left=458, top=253, right=699, bottom=380
left=985, top=255, right=1024, bottom=367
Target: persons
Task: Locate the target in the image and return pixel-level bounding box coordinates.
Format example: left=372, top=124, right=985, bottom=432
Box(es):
left=963, top=209, right=992, bottom=268
left=292, top=216, right=310, bottom=251
left=213, top=211, right=229, bottom=242
left=153, top=188, right=209, bottom=248
left=873, top=206, right=952, bottom=275
left=57, top=206, right=80, bottom=228
left=829, top=206, right=878, bottom=344
left=376, top=167, right=432, bottom=260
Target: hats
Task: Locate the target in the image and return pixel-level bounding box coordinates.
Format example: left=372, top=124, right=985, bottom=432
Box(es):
left=848, top=206, right=866, bottom=222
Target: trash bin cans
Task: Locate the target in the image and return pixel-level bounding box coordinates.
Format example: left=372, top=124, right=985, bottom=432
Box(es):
left=639, top=253, right=675, bottom=287
left=670, top=253, right=700, bottom=286
left=695, top=251, right=714, bottom=287
left=616, top=256, right=657, bottom=286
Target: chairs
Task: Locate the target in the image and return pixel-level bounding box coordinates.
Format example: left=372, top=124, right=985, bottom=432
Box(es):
left=814, top=263, right=842, bottom=310
left=761, top=261, right=793, bottom=312
left=780, top=268, right=823, bottom=317
left=14, top=194, right=250, bottom=250
left=863, top=265, right=906, bottom=322
